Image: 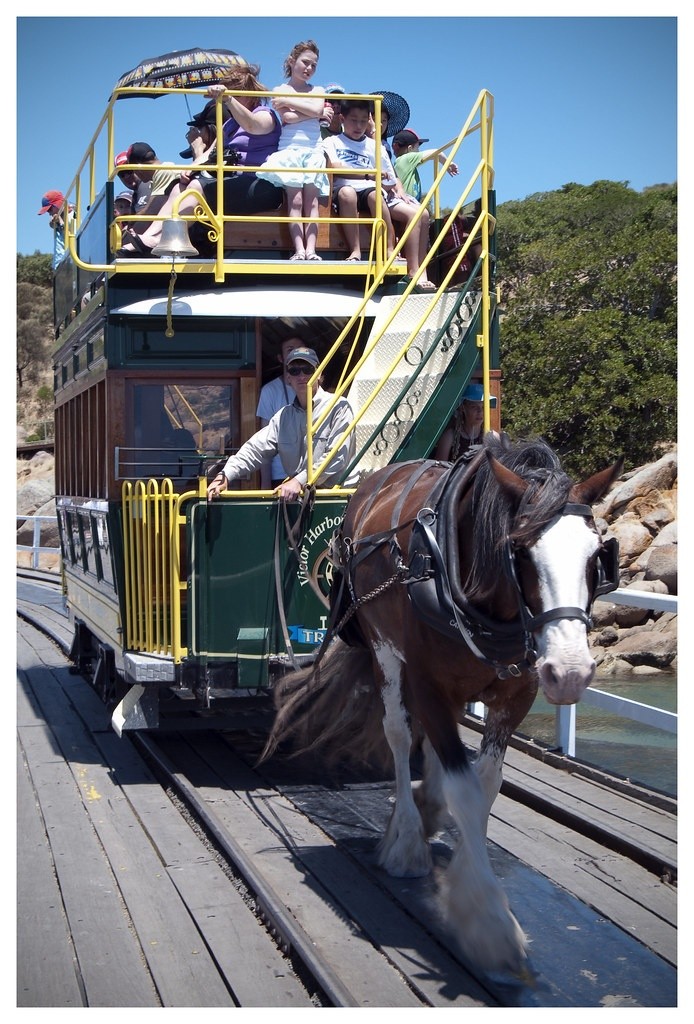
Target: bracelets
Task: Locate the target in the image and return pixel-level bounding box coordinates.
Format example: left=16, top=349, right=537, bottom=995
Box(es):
left=222, top=95, right=232, bottom=105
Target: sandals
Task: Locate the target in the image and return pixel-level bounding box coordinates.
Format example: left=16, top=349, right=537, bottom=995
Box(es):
left=306, top=253, right=322, bottom=261
left=289, top=253, right=306, bottom=260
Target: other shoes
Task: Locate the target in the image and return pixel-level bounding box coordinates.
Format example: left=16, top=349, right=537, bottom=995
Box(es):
left=408, top=276, right=436, bottom=289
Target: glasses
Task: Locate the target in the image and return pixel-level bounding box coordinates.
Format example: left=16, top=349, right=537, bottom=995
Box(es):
left=287, top=366, right=315, bottom=376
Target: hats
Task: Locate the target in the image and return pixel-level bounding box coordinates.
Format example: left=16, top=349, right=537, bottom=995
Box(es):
left=187, top=120, right=206, bottom=127
left=368, top=90, right=409, bottom=140
left=324, top=84, right=345, bottom=94
left=286, top=346, right=320, bottom=368
left=38, top=190, right=66, bottom=215
left=193, top=99, right=233, bottom=124
left=114, top=151, right=129, bottom=166
left=115, top=191, right=134, bottom=204
left=392, top=130, right=428, bottom=148
left=179, top=146, right=193, bottom=159
left=460, top=384, right=498, bottom=402
left=126, top=142, right=156, bottom=165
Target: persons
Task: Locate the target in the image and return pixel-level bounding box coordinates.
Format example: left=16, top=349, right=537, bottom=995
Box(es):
left=82, top=63, right=283, bottom=312
left=135, top=428, right=199, bottom=488
left=435, top=384, right=497, bottom=464
left=207, top=347, right=355, bottom=503
left=256, top=335, right=308, bottom=490
left=320, top=84, right=459, bottom=289
left=38, top=190, right=77, bottom=317
left=255, top=40, right=335, bottom=261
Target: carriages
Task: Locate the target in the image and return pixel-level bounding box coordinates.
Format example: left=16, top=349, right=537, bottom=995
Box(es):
left=50, top=86, right=620, bottom=997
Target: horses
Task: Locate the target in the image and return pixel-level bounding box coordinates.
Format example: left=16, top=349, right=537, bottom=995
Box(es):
left=253, top=434, right=624, bottom=986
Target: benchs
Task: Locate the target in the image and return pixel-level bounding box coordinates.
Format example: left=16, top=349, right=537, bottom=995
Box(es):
left=218, top=153, right=376, bottom=251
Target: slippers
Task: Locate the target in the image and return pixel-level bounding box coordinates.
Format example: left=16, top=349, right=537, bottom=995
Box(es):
left=345, top=255, right=362, bottom=261
left=117, top=235, right=161, bottom=258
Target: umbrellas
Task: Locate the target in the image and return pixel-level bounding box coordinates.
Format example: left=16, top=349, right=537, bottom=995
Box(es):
left=107, top=46, right=252, bottom=121
